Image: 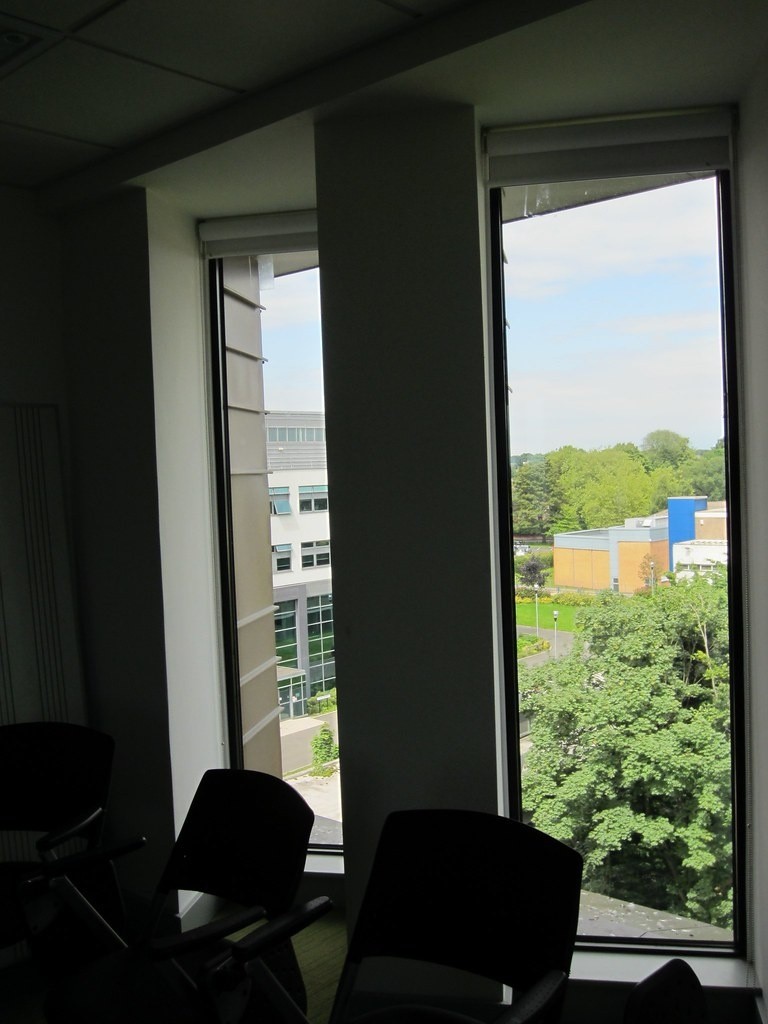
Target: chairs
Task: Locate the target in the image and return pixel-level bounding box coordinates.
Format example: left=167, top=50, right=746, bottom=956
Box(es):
left=0, top=721, right=148, bottom=969
left=328, top=809, right=584, bottom=1024
left=43, top=769, right=333, bottom=1024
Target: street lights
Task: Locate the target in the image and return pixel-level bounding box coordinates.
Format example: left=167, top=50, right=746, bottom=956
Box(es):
left=552, top=610, right=558, bottom=660
left=650, top=562, right=655, bottom=596
left=533, top=583, right=540, bottom=637
